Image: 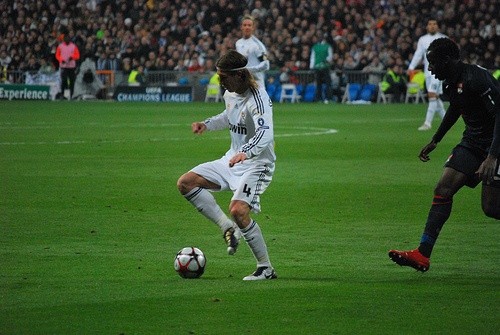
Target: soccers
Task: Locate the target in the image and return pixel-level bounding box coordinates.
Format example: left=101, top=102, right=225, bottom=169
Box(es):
left=173, top=247, right=207, bottom=279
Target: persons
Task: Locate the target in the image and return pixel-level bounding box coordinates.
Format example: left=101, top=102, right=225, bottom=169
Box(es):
left=177, top=50, right=276, bottom=281
left=56, top=35, right=80, bottom=100
left=407, top=18, right=448, bottom=130
left=409, top=65, right=429, bottom=103
left=235, top=15, right=269, bottom=86
left=208, top=72, right=220, bottom=95
left=389, top=38, right=500, bottom=273
left=309, top=30, right=333, bottom=103
left=128, top=65, right=144, bottom=84
left=0, top=0, right=500, bottom=83
left=382, top=65, right=406, bottom=103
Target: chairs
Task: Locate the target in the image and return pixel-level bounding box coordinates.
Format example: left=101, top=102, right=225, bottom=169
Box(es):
left=204, top=82, right=428, bottom=104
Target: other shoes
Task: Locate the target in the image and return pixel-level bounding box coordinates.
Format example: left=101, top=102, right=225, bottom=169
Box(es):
left=417, top=121, right=432, bottom=131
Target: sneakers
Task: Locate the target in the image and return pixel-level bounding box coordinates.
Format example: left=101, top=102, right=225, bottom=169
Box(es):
left=242, top=265, right=275, bottom=281
left=222, top=221, right=243, bottom=255
left=389, top=247, right=431, bottom=273
